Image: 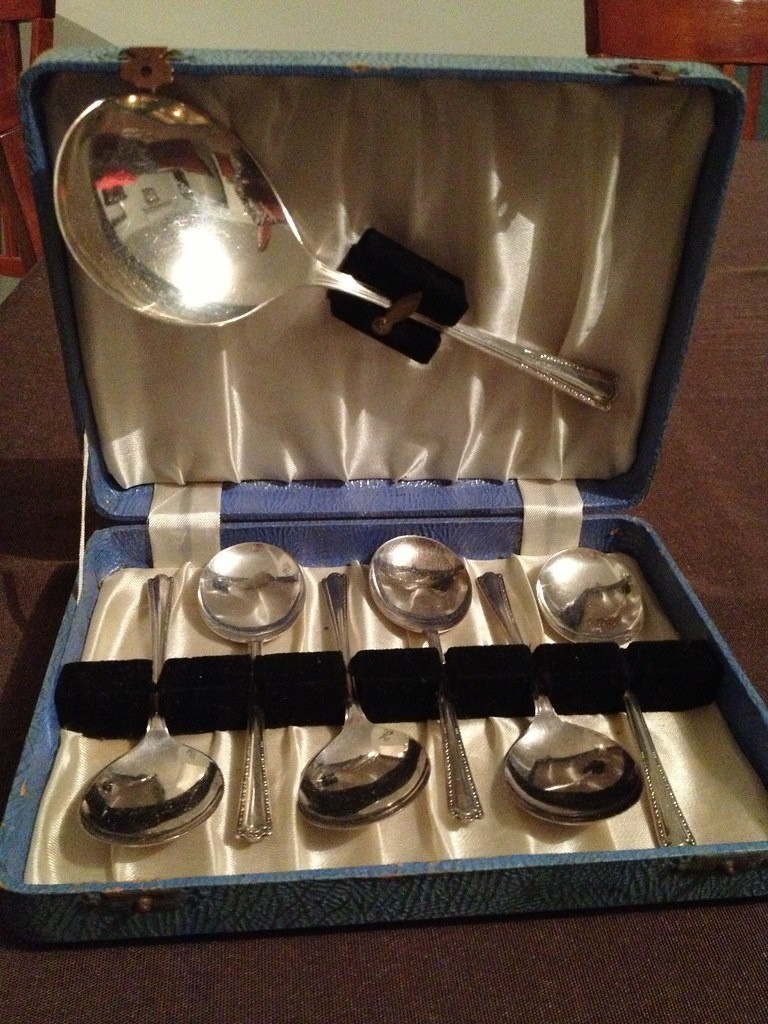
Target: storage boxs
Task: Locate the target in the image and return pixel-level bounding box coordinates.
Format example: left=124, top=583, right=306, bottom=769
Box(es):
left=0, top=48, right=768, bottom=949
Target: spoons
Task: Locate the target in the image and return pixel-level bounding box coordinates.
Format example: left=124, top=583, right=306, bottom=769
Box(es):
left=53, top=90, right=618, bottom=416
left=196, top=542, right=306, bottom=844
left=541, top=550, right=696, bottom=849
left=368, top=533, right=483, bottom=828
left=294, top=568, right=434, bottom=834
left=473, top=572, right=646, bottom=826
left=80, top=572, right=222, bottom=851
